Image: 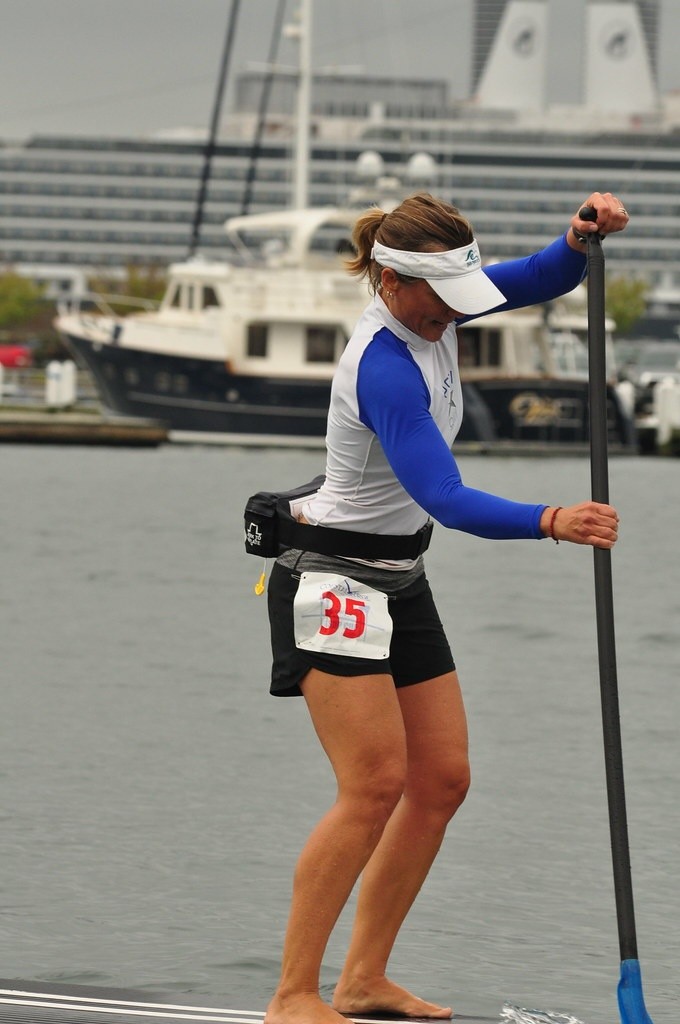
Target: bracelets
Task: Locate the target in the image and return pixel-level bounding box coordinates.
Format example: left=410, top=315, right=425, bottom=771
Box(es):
left=571, top=228, right=607, bottom=244
left=548, top=507, right=563, bottom=544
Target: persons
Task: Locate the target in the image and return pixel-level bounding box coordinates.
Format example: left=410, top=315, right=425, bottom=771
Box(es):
left=265, top=190, right=631, bottom=1024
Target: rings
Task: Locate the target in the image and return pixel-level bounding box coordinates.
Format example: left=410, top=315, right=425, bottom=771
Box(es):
left=619, top=207, right=627, bottom=215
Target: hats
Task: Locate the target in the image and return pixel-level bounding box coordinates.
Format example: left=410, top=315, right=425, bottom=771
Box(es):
left=370, top=235, right=506, bottom=315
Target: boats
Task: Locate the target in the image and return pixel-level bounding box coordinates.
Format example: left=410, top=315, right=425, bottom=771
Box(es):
left=54, top=204, right=637, bottom=455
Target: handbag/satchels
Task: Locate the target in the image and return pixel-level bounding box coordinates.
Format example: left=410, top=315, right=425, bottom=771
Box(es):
left=243, top=476, right=326, bottom=558
